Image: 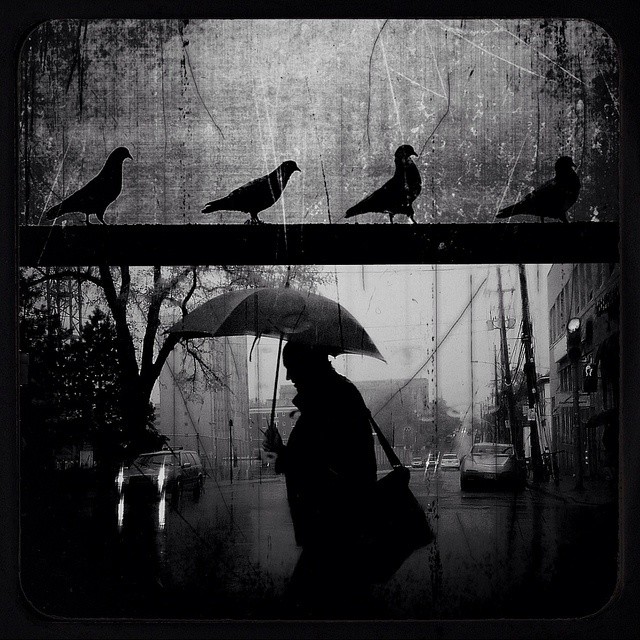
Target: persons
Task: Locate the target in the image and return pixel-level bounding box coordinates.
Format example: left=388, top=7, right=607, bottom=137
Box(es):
left=263, top=341, right=380, bottom=638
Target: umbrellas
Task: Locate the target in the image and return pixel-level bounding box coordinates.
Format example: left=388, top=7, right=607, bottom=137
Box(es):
left=159, top=279, right=387, bottom=453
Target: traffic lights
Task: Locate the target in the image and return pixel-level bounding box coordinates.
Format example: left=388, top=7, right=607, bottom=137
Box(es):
left=566, top=317, right=584, bottom=365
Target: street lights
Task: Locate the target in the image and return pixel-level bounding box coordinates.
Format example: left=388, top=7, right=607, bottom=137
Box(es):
left=470, top=360, right=501, bottom=376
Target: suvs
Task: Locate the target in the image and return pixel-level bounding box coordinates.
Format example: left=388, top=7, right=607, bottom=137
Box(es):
left=115, top=450, right=205, bottom=500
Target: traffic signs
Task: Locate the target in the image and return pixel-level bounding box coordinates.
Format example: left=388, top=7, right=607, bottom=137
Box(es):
left=486, top=317, right=526, bottom=467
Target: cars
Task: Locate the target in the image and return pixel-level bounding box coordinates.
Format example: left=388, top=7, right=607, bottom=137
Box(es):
left=412, top=457, right=424, bottom=468
left=427, top=457, right=439, bottom=467
left=440, top=453, right=460, bottom=469
left=459, top=442, right=523, bottom=494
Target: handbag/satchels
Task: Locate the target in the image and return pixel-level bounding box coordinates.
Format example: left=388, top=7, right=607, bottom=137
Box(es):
left=339, top=465, right=439, bottom=594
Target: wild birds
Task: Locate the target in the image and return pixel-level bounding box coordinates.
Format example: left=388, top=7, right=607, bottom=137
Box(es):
left=46, top=145, right=134, bottom=226
left=343, top=143, right=423, bottom=224
left=201, top=160, right=302, bottom=225
left=495, top=154, right=582, bottom=225
